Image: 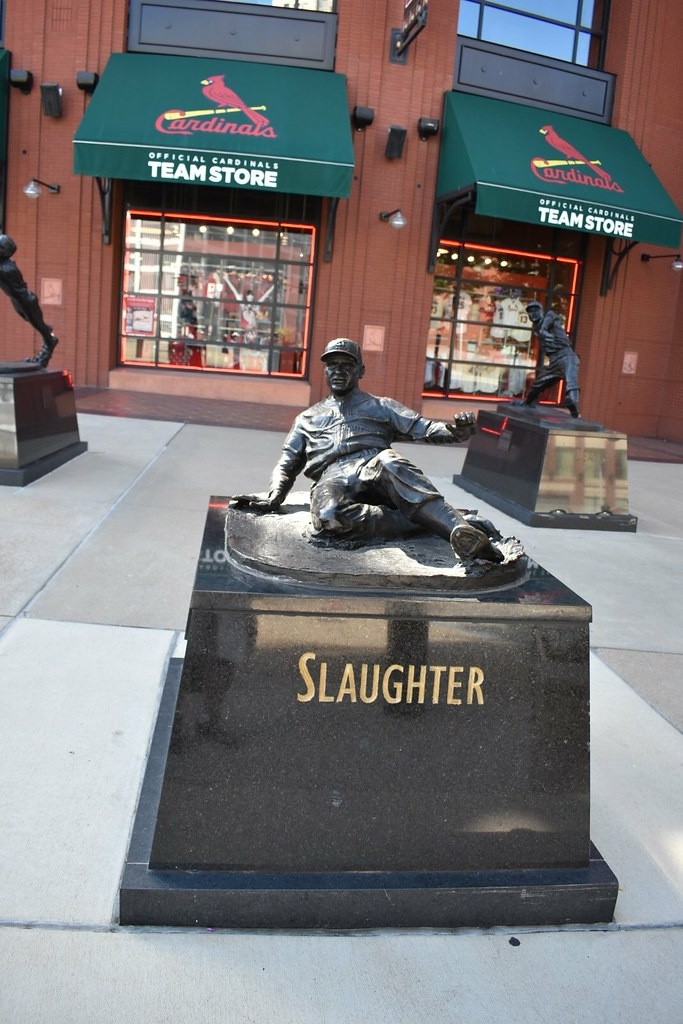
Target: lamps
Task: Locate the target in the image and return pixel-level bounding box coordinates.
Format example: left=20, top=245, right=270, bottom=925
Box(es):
left=418, top=117, right=439, bottom=141
left=24, top=179, right=60, bottom=200
left=380, top=208, right=407, bottom=230
left=641, top=254, right=683, bottom=272
left=10, top=69, right=33, bottom=94
left=76, top=71, right=98, bottom=93
left=352, top=106, right=374, bottom=132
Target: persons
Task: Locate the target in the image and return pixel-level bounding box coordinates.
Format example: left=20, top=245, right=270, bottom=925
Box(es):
left=0, top=233, right=59, bottom=367
left=223, top=272, right=274, bottom=338
left=231, top=337, right=506, bottom=564
left=511, top=302, right=582, bottom=418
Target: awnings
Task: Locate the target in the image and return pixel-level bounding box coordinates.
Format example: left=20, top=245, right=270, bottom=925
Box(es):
left=72, top=52, right=355, bottom=198
left=436, top=90, right=683, bottom=250
